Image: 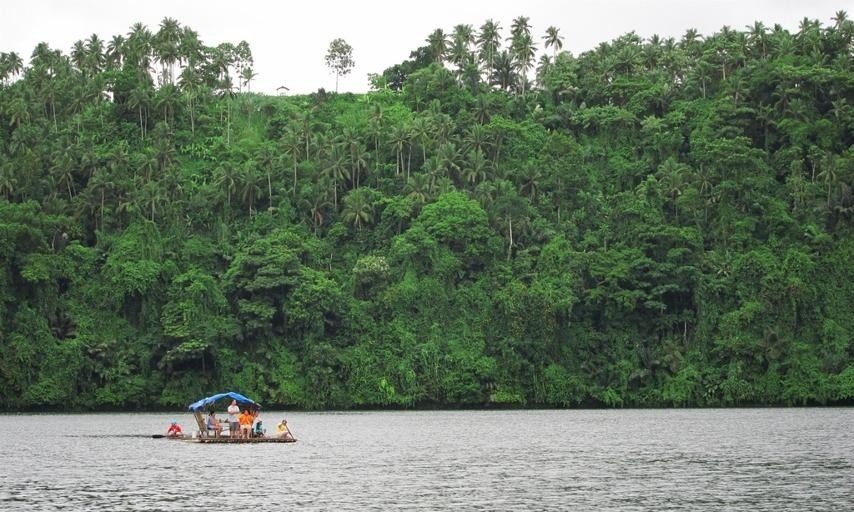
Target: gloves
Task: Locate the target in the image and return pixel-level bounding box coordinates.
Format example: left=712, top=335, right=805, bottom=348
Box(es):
left=166, top=391, right=297, bottom=443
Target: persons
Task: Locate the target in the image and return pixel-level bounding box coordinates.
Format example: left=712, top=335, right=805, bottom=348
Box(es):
left=227, top=398, right=240, bottom=438
left=255, top=420, right=266, bottom=437
left=274, top=419, right=288, bottom=439
left=236, top=409, right=253, bottom=439
left=208, top=410, right=220, bottom=437
left=167, top=418, right=183, bottom=436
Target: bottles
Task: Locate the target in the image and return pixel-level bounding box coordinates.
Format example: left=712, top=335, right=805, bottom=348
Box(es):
left=256, top=418, right=264, bottom=423
left=170, top=418, right=176, bottom=424
